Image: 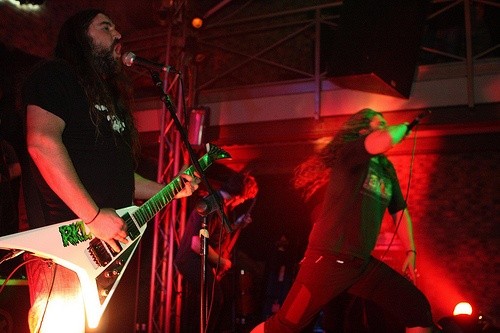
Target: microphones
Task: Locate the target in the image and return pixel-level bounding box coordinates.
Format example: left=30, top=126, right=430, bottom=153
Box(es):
left=408, top=108, right=431, bottom=131
left=121, top=48, right=178, bottom=74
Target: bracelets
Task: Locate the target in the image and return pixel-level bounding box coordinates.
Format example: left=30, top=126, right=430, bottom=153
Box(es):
left=405, top=250, right=416, bottom=255
left=84, top=207, right=101, bottom=225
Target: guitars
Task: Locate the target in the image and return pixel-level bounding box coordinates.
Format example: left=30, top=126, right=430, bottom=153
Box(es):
left=212, top=196, right=257, bottom=281
left=0, top=141, right=233, bottom=331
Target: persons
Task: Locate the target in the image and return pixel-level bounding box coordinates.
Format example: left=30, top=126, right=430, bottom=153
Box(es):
left=249, top=108, right=432, bottom=333
left=0, top=89, right=22, bottom=297
left=174, top=173, right=258, bottom=333
left=19, top=8, right=203, bottom=333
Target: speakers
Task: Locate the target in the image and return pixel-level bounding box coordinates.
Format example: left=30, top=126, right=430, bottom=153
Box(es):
left=186, top=105, right=210, bottom=149
left=0, top=279, right=32, bottom=333
